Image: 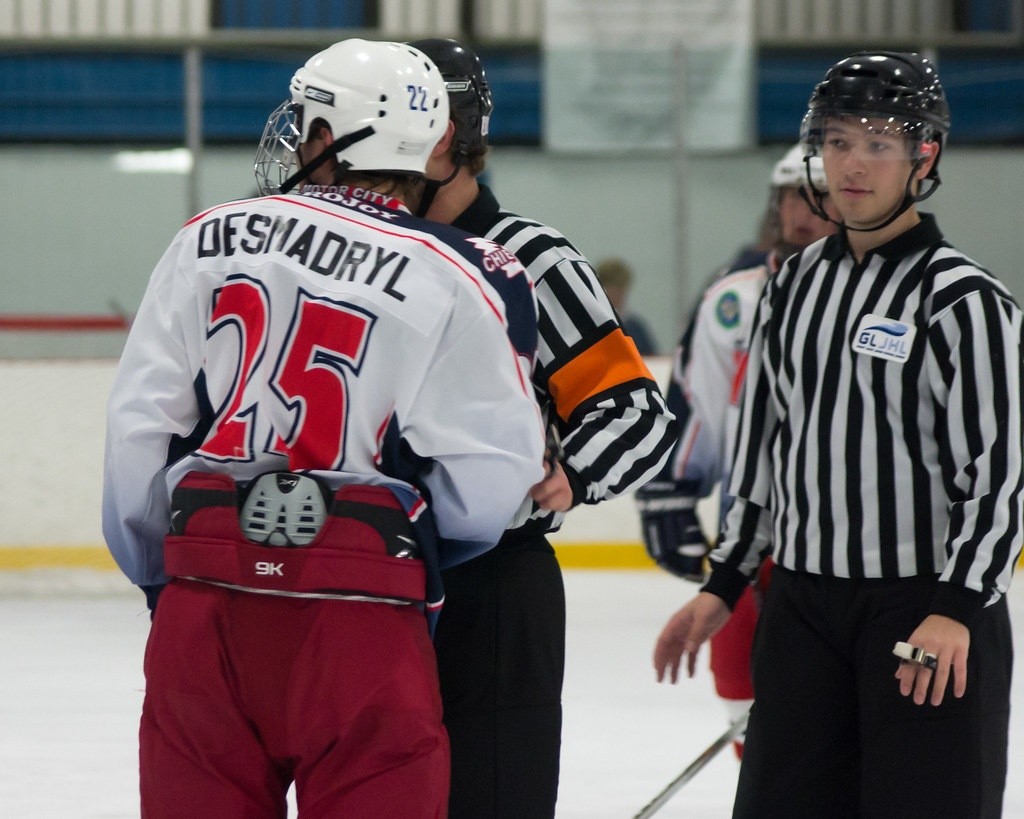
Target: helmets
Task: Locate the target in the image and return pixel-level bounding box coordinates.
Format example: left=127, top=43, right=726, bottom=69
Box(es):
left=769, top=140, right=829, bottom=193
left=250, top=38, right=451, bottom=202
left=795, top=43, right=951, bottom=170
left=396, top=38, right=496, bottom=166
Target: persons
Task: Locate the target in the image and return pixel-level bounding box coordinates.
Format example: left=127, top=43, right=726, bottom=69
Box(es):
left=397, top=39, right=674, bottom=818
left=653, top=48, right=1024, bottom=819
left=591, top=255, right=653, bottom=351
left=636, top=137, right=848, bottom=752
left=103, top=37, right=544, bottom=819
